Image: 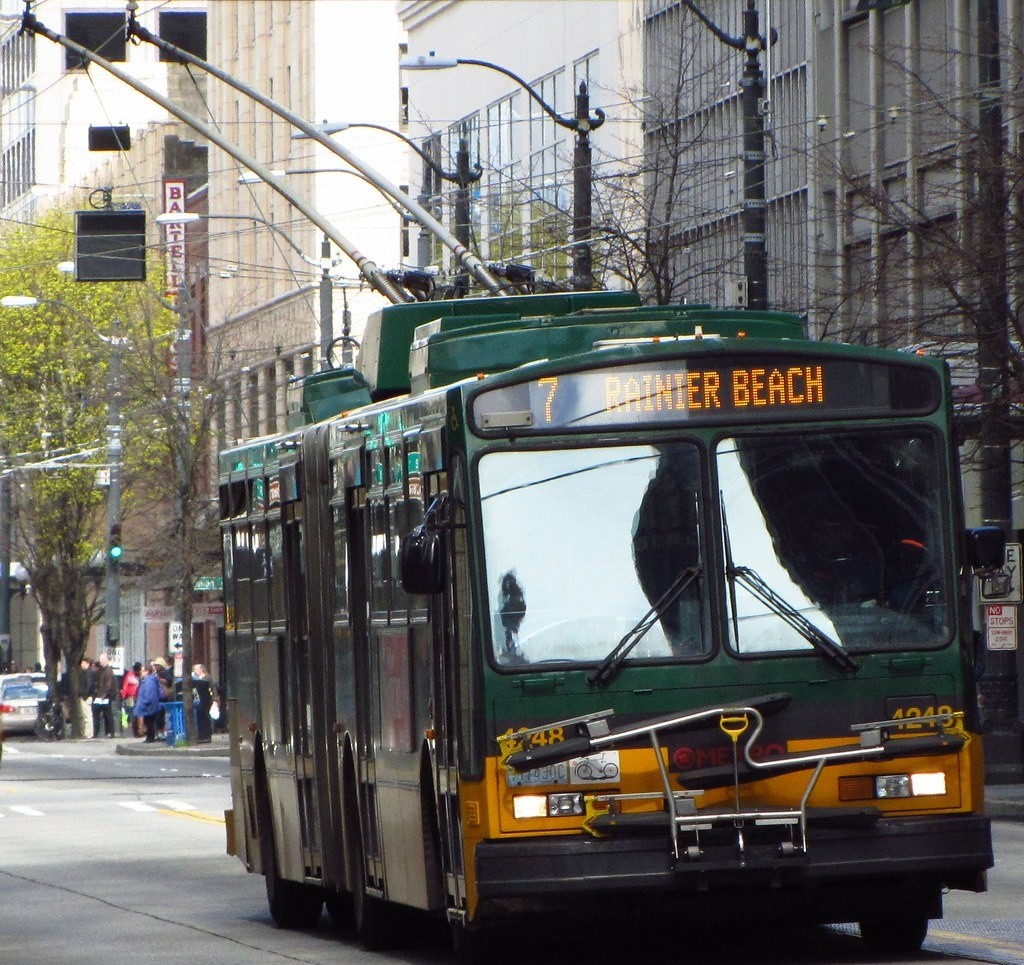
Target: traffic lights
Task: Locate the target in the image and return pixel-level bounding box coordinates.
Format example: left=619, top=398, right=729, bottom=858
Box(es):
left=110, top=522, right=122, bottom=561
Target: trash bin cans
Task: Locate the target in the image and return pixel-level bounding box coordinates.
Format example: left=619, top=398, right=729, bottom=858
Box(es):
left=160, top=702, right=198, bottom=747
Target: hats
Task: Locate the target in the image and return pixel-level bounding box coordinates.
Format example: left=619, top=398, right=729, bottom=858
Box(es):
left=152, top=657, right=167, bottom=669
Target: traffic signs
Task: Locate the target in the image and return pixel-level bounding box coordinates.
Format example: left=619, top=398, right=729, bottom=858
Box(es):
left=168, top=621, right=184, bottom=654
left=107, top=646, right=125, bottom=676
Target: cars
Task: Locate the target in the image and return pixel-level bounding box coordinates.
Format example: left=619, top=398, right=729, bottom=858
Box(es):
left=0, top=672, right=53, bottom=733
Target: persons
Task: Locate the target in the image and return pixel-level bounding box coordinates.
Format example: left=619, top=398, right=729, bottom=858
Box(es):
left=77, top=652, right=117, bottom=738
left=133, top=666, right=160, bottom=742
left=12, top=661, right=63, bottom=682
left=192, top=664, right=219, bottom=708
left=123, top=662, right=145, bottom=737
left=152, top=657, right=172, bottom=738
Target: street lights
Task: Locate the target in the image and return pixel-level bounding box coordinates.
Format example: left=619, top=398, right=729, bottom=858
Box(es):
left=399, top=51, right=604, bottom=294
left=155, top=210, right=334, bottom=374
left=289, top=123, right=483, bottom=299
left=0, top=295, right=123, bottom=736
left=59, top=260, right=193, bottom=684
left=238, top=166, right=443, bottom=276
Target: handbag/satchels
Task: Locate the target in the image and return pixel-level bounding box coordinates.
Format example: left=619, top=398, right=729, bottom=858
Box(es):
left=209, top=701, right=220, bottom=720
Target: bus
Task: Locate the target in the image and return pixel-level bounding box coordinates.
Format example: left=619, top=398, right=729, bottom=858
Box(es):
left=223, top=291, right=1005, bottom=965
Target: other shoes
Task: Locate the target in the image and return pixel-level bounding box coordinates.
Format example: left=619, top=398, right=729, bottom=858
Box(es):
left=142, top=739, right=155, bottom=743
left=196, top=738, right=211, bottom=744
